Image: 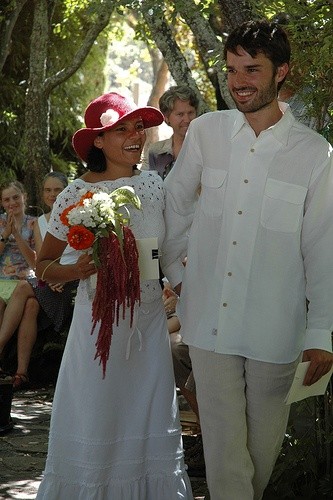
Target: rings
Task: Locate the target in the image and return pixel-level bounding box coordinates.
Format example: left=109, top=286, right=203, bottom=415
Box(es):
left=168, top=308, right=172, bottom=314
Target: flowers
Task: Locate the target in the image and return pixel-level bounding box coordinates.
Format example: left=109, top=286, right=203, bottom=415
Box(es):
left=100, top=108, right=120, bottom=127
left=61, top=186, right=143, bottom=379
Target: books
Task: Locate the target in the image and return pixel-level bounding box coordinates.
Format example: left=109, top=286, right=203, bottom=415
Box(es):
left=284, top=361, right=332, bottom=405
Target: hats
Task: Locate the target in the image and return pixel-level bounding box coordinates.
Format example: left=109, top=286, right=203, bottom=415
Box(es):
left=72, top=92, right=163, bottom=163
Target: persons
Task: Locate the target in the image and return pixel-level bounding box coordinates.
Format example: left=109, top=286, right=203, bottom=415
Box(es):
left=160, top=19, right=333, bottom=500
left=36, top=92, right=194, bottom=500
left=0, top=70, right=322, bottom=435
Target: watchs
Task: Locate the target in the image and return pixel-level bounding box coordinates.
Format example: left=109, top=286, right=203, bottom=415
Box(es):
left=0, top=234, right=9, bottom=243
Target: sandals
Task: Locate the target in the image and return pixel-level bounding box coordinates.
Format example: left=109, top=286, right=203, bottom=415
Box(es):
left=14, top=373, right=30, bottom=391
